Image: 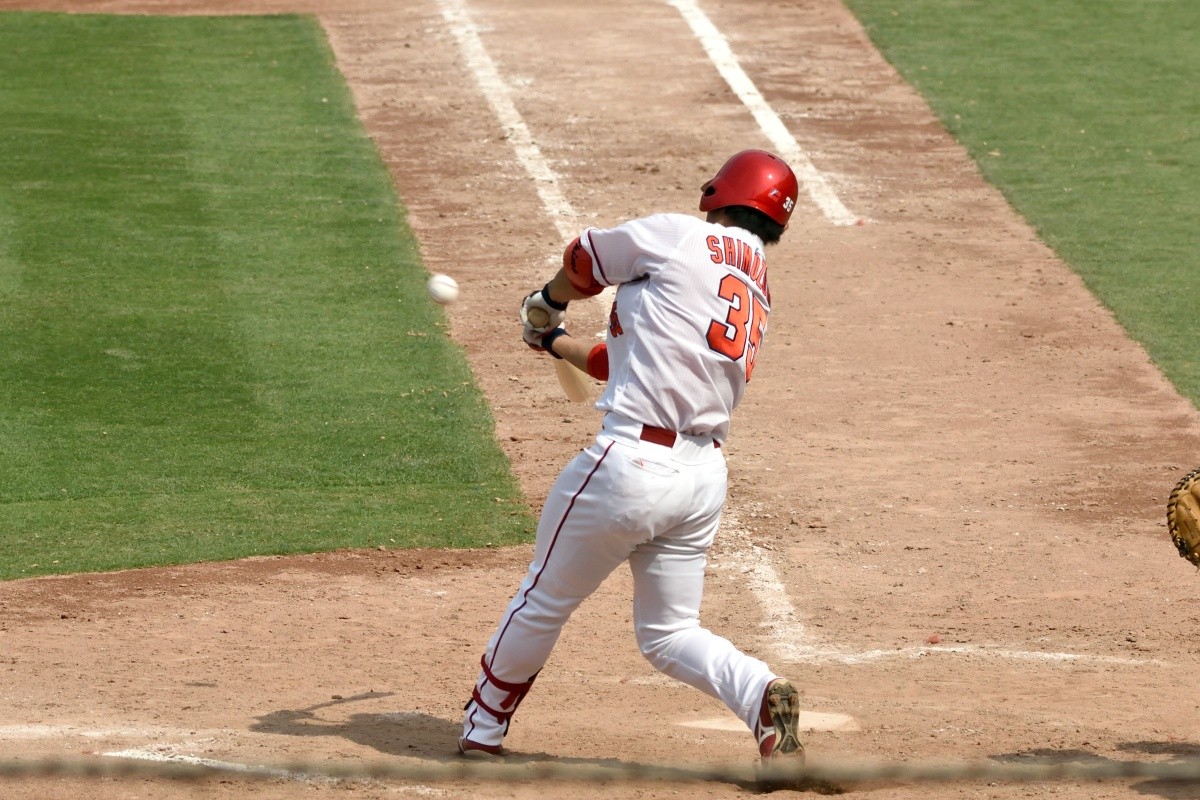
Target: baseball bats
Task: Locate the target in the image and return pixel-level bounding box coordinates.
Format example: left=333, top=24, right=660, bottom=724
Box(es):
left=528, top=306, right=592, bottom=404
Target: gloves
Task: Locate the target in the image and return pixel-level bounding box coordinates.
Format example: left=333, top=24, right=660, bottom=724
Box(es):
left=520, top=283, right=568, bottom=333
left=521, top=321, right=572, bottom=360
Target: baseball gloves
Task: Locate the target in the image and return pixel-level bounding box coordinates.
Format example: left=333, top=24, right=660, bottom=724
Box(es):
left=1165, top=468, right=1200, bottom=571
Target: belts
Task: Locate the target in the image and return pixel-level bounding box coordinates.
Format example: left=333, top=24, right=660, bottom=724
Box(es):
left=640, top=425, right=720, bottom=448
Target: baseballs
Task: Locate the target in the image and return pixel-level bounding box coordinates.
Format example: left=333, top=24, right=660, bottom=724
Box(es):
left=429, top=274, right=459, bottom=305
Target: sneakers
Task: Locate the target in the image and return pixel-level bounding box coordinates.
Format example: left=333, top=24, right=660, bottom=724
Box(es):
left=457, top=736, right=504, bottom=757
left=753, top=677, right=808, bottom=794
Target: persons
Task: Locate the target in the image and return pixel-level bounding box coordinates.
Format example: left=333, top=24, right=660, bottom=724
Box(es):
left=459, top=149, right=805, bottom=785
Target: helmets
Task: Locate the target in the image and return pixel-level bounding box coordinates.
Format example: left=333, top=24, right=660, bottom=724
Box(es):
left=699, top=149, right=799, bottom=226
